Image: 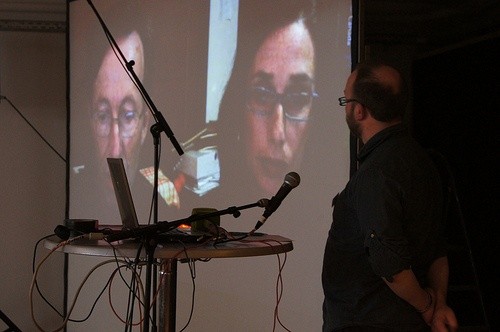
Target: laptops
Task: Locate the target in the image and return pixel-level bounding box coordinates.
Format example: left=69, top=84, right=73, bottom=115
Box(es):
left=106, top=157, right=213, bottom=239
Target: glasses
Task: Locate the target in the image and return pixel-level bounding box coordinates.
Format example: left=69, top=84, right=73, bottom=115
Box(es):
left=338, top=97, right=365, bottom=111
left=247, top=85, right=321, bottom=121
left=88, top=108, right=145, bottom=138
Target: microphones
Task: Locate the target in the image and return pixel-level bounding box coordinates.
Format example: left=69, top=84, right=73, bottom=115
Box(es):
left=254, top=171, right=300, bottom=230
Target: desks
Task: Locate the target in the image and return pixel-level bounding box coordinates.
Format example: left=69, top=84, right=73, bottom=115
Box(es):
left=43, top=232, right=294, bottom=332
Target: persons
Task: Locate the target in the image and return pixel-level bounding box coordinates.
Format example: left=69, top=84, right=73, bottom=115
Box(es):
left=195, top=2, right=337, bottom=210
left=322, top=63, right=457, bottom=332
left=70, top=22, right=199, bottom=226
